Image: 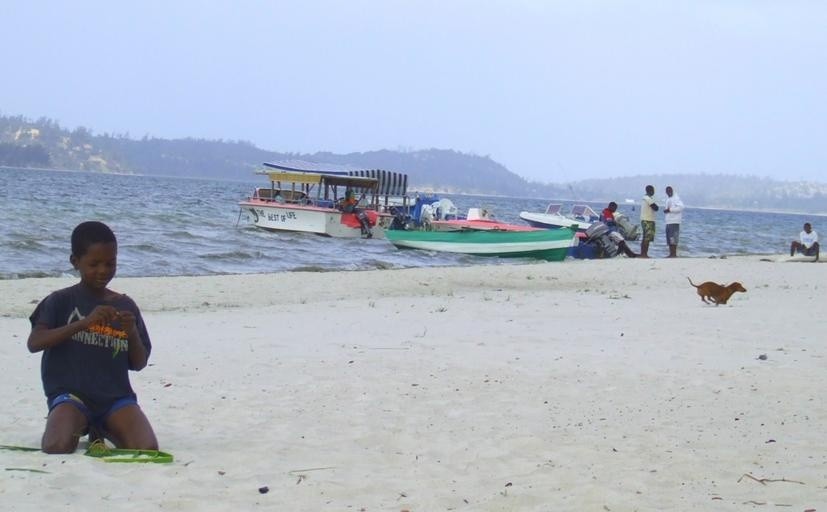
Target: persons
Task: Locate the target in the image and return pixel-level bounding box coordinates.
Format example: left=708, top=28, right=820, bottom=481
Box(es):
left=639, top=184, right=659, bottom=258
left=664, top=186, right=684, bottom=259
left=599, top=201, right=640, bottom=257
left=26, top=220, right=159, bottom=454
left=790, top=223, right=821, bottom=261
left=275, top=187, right=369, bottom=210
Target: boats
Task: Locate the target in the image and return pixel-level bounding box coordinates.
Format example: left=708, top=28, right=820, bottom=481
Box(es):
left=236, top=168, right=408, bottom=240
left=383, top=194, right=640, bottom=263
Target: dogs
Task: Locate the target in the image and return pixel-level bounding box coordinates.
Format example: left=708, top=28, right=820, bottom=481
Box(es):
left=686, top=275, right=746, bottom=306
